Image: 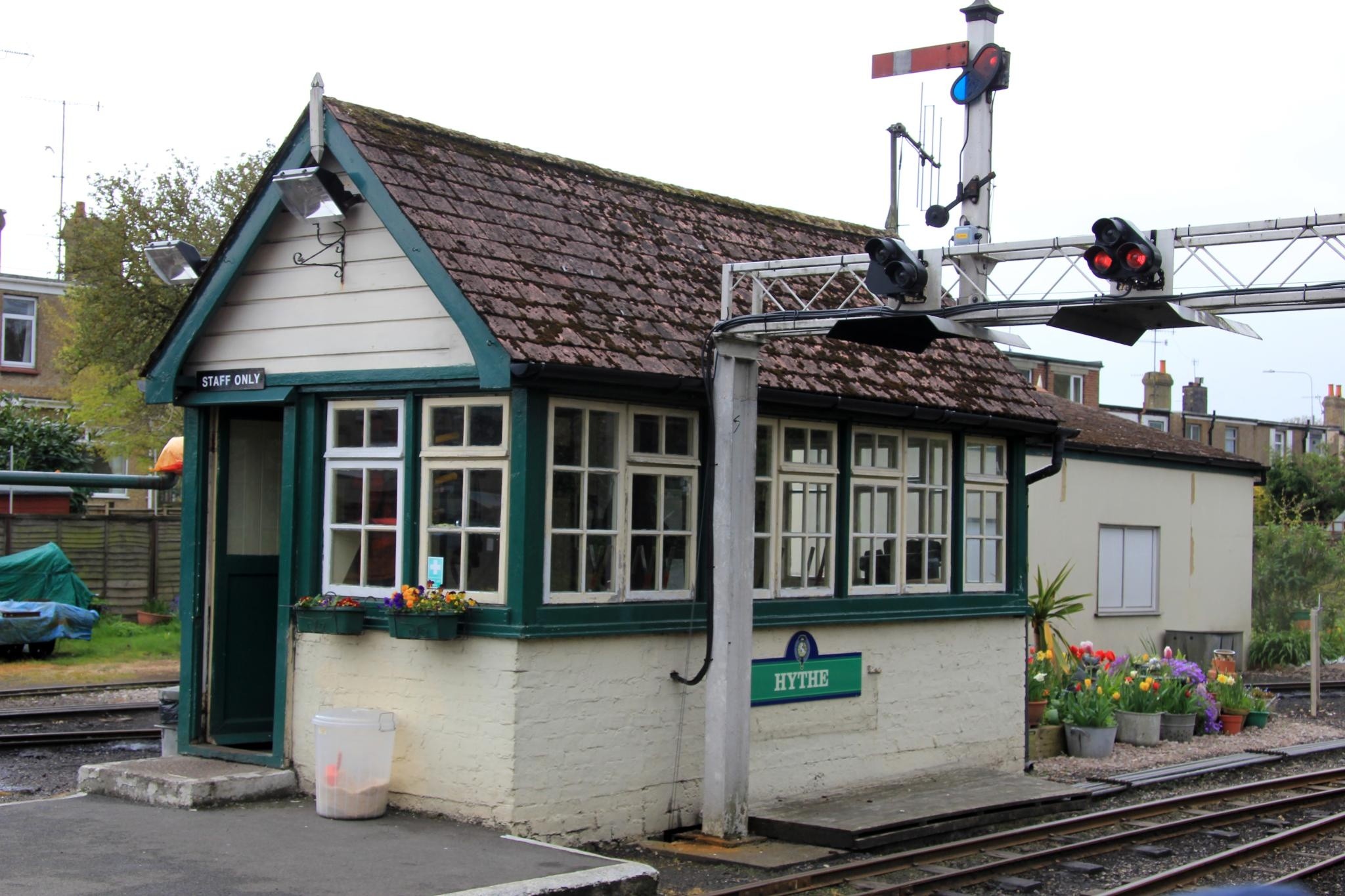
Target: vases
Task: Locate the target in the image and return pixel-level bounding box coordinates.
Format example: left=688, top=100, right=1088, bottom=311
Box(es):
left=1218, top=714, right=1242, bottom=736
left=1063, top=722, right=1119, bottom=760
left=1211, top=648, right=1238, bottom=682
left=1244, top=709, right=1270, bottom=729
left=294, top=605, right=367, bottom=634
left=1113, top=708, right=1166, bottom=748
left=1158, top=710, right=1198, bottom=745
left=1220, top=707, right=1251, bottom=736
left=1207, top=693, right=1217, bottom=700
left=384, top=609, right=466, bottom=641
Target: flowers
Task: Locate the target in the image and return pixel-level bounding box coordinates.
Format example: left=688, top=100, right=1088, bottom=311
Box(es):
left=376, top=579, right=484, bottom=614
left=290, top=595, right=363, bottom=607
left=1017, top=639, right=1221, bottom=729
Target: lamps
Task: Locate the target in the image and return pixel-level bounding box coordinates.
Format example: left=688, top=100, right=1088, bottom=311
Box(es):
left=271, top=165, right=367, bottom=226
left=143, top=240, right=210, bottom=287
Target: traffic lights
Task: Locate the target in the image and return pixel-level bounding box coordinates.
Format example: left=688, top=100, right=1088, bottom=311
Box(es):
left=1082, top=218, right=1161, bottom=282
left=864, top=236, right=929, bottom=296
